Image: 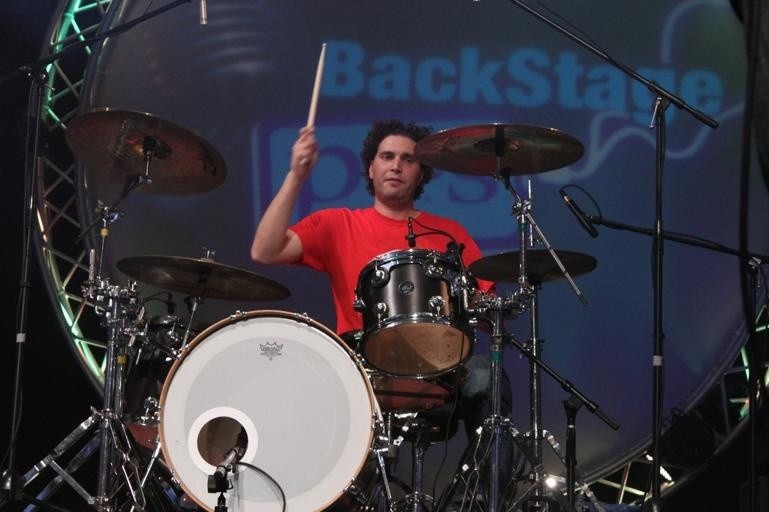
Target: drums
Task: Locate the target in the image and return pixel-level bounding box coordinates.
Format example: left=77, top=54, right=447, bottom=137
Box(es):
left=104, top=317, right=205, bottom=451
left=158, top=310, right=377, bottom=511
left=338, top=329, right=463, bottom=415
left=353, top=248, right=477, bottom=379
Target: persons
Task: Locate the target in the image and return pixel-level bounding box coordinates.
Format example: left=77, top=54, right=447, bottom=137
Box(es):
left=248, top=115, right=516, bottom=507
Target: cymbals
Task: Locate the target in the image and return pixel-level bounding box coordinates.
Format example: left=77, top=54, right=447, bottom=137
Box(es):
left=414, top=123, right=585, bottom=176
left=65, top=108, right=227, bottom=195
left=116, top=255, right=291, bottom=300
left=468, top=249, right=598, bottom=283
